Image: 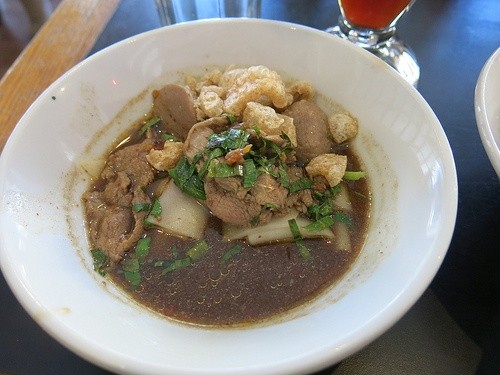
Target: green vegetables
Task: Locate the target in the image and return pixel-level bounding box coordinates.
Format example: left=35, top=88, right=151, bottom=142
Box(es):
left=89, top=111, right=366, bottom=287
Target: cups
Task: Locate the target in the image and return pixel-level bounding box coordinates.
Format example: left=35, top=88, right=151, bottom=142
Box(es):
left=155, top=0, right=261, bottom=27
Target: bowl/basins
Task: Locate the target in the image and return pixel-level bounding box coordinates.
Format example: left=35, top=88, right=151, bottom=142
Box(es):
left=474, top=48, right=500, bottom=178
left=0, top=17, right=457, bottom=375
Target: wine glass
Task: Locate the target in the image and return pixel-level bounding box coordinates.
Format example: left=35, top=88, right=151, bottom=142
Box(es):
left=324, top=0, right=420, bottom=86
